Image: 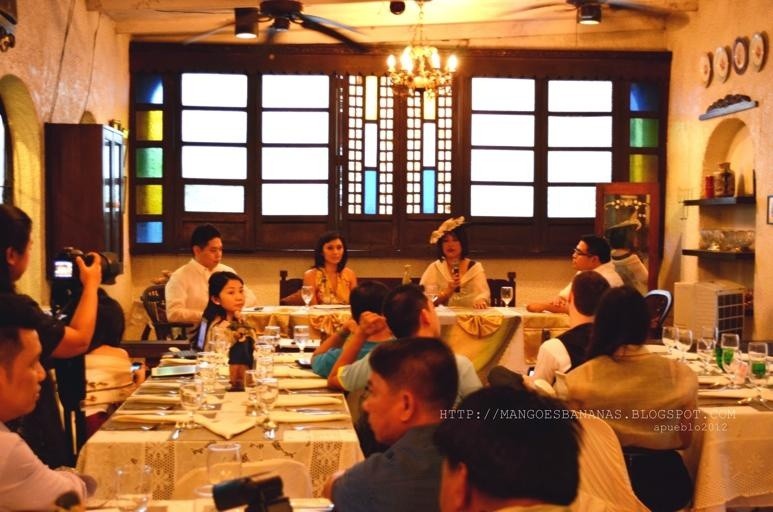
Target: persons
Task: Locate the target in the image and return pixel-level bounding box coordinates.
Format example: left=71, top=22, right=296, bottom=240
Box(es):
left=189, top=270, right=253, bottom=353
left=523, top=234, right=620, bottom=315
left=299, top=229, right=358, bottom=305
left=0, top=294, right=87, bottom=512
left=419, top=225, right=492, bottom=310
left=306, top=278, right=698, bottom=512
left=0, top=203, right=102, bottom=469
left=164, top=225, right=258, bottom=338
left=78, top=291, right=143, bottom=416
left=604, top=223, right=648, bottom=295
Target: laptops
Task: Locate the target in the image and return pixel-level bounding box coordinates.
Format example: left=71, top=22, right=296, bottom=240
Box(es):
left=161, top=318, right=208, bottom=359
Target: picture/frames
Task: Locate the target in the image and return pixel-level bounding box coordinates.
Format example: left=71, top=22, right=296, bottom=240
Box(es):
left=766, top=194, right=772, bottom=224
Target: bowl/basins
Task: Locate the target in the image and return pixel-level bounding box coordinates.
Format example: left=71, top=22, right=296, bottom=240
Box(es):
left=700, top=229, right=754, bottom=251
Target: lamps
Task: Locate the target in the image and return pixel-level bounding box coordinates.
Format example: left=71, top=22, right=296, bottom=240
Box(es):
left=384, top=0, right=459, bottom=101
left=227, top=6, right=261, bottom=39
left=274, top=15, right=291, bottom=32
left=574, top=4, right=603, bottom=25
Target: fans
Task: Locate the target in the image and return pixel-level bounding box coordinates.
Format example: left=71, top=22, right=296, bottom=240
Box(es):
left=156, top=0, right=374, bottom=51
left=497, top=0, right=691, bottom=23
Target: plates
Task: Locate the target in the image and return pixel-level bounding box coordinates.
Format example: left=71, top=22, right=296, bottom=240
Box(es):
left=288, top=497, right=333, bottom=511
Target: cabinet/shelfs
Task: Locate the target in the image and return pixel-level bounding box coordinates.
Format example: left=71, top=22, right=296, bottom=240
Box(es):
left=681, top=195, right=756, bottom=260
left=43, top=116, right=127, bottom=285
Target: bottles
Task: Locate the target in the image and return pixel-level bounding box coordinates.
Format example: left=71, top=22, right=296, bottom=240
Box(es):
left=703, top=162, right=735, bottom=196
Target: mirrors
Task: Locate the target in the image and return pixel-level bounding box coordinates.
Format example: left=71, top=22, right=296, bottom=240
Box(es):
left=594, top=179, right=662, bottom=299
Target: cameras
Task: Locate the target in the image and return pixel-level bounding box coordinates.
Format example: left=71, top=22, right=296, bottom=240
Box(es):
left=47, top=246, right=123, bottom=286
left=211, top=472, right=293, bottom=512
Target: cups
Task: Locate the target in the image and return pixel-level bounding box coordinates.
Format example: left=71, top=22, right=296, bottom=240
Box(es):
left=115, top=465, right=152, bottom=512
left=424, top=285, right=437, bottom=308
left=192, top=487, right=214, bottom=512
left=205, top=443, right=244, bottom=487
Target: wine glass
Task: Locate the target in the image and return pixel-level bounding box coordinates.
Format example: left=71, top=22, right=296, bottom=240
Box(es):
left=662, top=326, right=772, bottom=410
left=500, top=286, right=513, bottom=311
left=301, top=285, right=313, bottom=311
left=101, top=327, right=352, bottom=441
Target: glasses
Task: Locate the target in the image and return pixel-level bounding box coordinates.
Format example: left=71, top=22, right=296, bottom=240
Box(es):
left=572, top=249, right=592, bottom=256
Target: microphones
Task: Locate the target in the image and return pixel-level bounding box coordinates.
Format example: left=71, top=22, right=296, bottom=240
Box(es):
left=451, top=258, right=460, bottom=292
